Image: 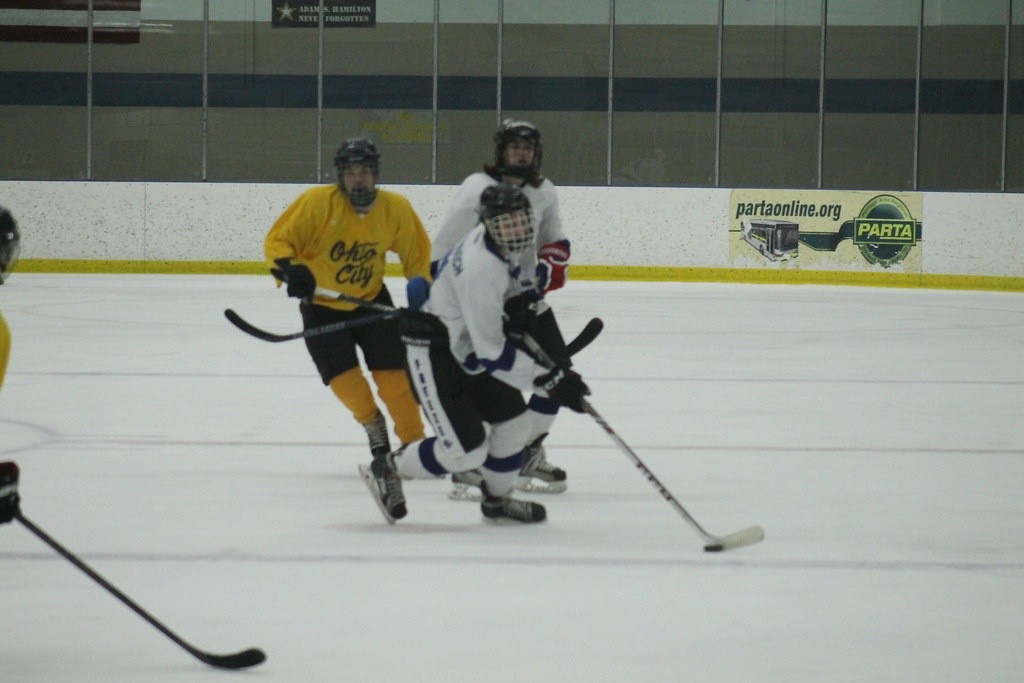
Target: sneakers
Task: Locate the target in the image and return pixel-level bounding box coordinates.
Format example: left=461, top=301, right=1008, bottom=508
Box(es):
left=522, top=445, right=567, bottom=494
left=447, top=471, right=482, bottom=501
left=479, top=481, right=547, bottom=527
left=361, top=411, right=393, bottom=456
left=358, top=454, right=407, bottom=525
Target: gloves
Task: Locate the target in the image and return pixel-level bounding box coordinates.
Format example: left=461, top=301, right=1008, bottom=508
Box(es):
left=534, top=368, right=591, bottom=412
left=0, top=462, right=20, bottom=522
left=286, top=265, right=317, bottom=302
left=506, top=290, right=539, bottom=334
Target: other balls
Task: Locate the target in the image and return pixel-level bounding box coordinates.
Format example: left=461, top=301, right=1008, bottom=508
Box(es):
left=704, top=543, right=721, bottom=553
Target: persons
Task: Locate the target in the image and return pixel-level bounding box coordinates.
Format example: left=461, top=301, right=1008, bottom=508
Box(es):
left=262, top=136, right=433, bottom=460
left=429, top=117, right=572, bottom=494
left=0, top=205, right=21, bottom=526
left=356, top=183, right=593, bottom=526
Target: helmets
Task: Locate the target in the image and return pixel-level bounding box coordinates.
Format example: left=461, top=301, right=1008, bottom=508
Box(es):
left=480, top=185, right=536, bottom=255
left=0, top=204, right=22, bottom=284
left=335, top=138, right=379, bottom=206
left=496, top=121, right=543, bottom=177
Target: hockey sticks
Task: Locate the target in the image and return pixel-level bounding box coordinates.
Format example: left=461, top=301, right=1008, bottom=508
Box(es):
left=500, top=309, right=767, bottom=552
left=223, top=306, right=405, bottom=343
left=269, top=264, right=605, bottom=357
left=15, top=512, right=267, bottom=670
